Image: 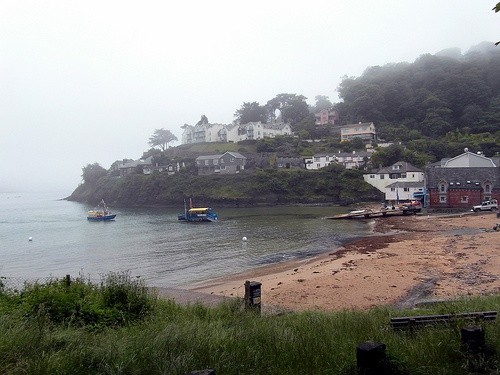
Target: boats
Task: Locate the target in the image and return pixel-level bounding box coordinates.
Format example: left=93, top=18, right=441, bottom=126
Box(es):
left=86, top=200, right=116, bottom=221
left=178, top=198, right=218, bottom=223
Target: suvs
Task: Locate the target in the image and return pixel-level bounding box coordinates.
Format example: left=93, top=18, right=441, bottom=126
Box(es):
left=472, top=199, right=498, bottom=213
